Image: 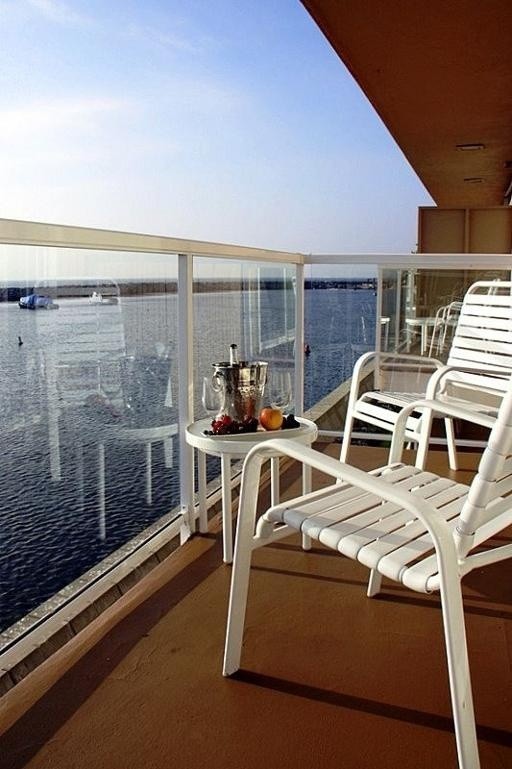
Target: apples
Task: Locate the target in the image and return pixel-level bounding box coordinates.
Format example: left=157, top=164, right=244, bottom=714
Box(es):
left=261, top=408, right=284, bottom=431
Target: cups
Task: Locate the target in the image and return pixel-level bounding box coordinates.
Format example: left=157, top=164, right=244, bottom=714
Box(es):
left=268, top=371, right=293, bottom=413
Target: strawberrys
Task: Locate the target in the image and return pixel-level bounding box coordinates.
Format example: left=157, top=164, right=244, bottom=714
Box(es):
left=212, top=420, right=222, bottom=431
left=220, top=414, right=231, bottom=427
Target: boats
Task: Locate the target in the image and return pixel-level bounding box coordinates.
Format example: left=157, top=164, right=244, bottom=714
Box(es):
left=304, top=343, right=311, bottom=354
left=89, top=290, right=120, bottom=305
left=17, top=293, right=60, bottom=311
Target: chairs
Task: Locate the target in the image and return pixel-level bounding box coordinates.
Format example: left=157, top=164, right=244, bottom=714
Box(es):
left=25, top=277, right=143, bottom=483
left=220, top=280, right=512, bottom=769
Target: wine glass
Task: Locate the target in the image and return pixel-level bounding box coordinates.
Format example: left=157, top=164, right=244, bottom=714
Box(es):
left=101, top=362, right=122, bottom=404
left=202, top=376, right=226, bottom=422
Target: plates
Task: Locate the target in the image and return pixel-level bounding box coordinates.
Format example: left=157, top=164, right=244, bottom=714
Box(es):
left=200, top=422, right=308, bottom=438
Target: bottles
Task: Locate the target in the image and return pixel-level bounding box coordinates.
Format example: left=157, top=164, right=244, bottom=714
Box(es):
left=229, top=344, right=240, bottom=368
left=162, top=340, right=177, bottom=360
